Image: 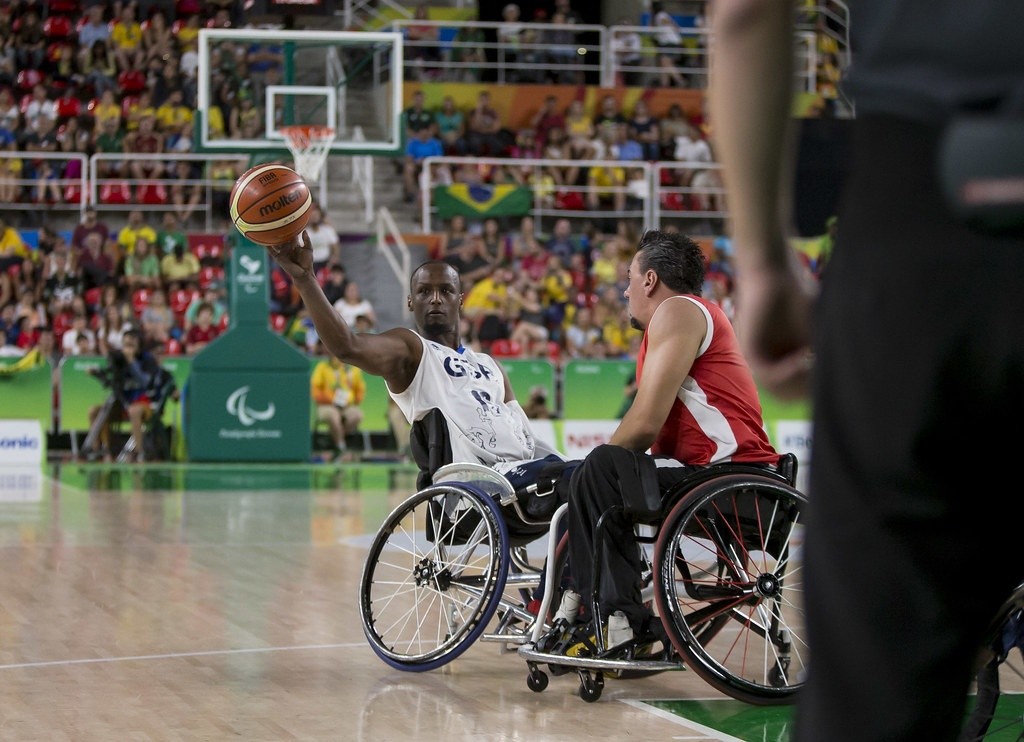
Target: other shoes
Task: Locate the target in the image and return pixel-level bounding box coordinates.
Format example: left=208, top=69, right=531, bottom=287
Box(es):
left=332, top=448, right=352, bottom=464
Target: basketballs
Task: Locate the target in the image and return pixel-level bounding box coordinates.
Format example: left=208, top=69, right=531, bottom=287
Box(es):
left=228, top=162, right=314, bottom=248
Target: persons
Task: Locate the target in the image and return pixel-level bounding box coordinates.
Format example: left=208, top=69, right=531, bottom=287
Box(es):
left=0, top=0, right=746, bottom=359
left=89, top=317, right=162, bottom=463
left=707, top=1, right=1024, bottom=741
left=310, top=356, right=366, bottom=460
left=262, top=226, right=642, bottom=625
left=530, top=230, right=780, bottom=654
